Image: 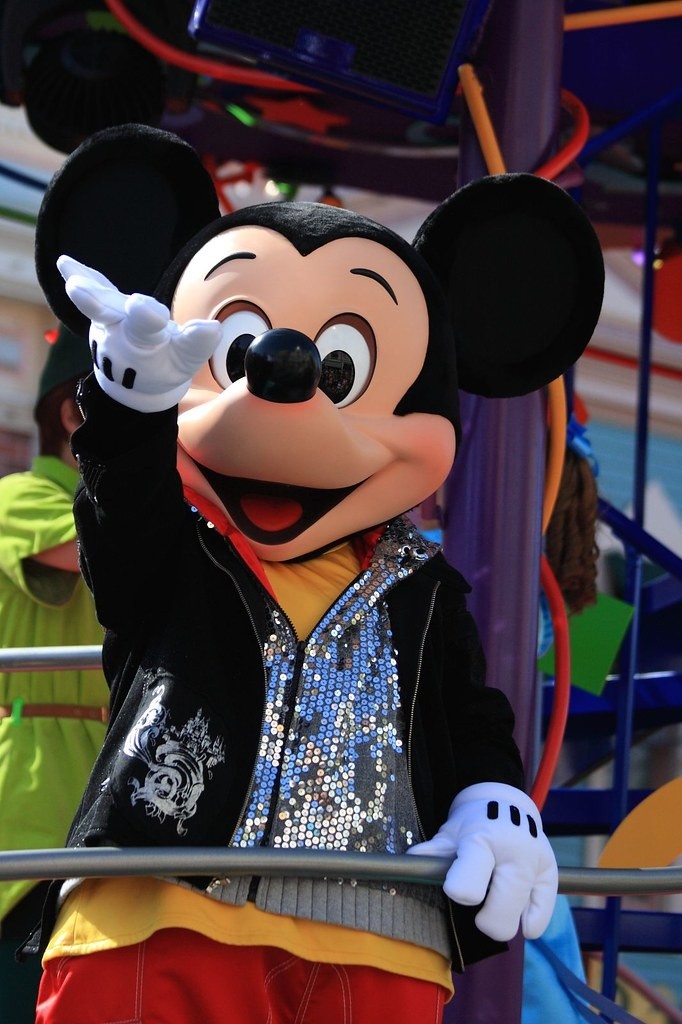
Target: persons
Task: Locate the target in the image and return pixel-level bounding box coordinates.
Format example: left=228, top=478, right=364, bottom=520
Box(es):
left=417, top=416, right=602, bottom=1023
left=0, top=323, right=113, bottom=1024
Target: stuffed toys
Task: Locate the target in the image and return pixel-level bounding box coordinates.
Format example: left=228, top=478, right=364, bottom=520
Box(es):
left=33, top=125, right=604, bottom=1024
